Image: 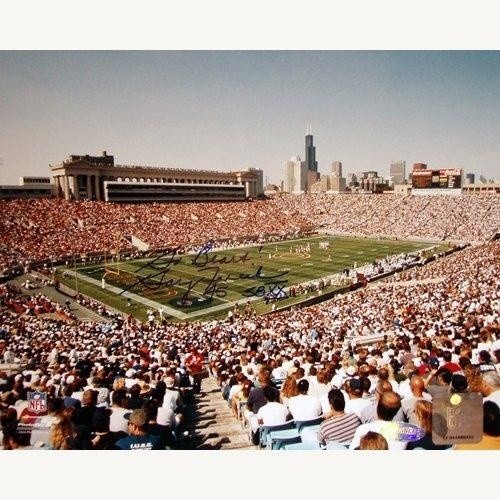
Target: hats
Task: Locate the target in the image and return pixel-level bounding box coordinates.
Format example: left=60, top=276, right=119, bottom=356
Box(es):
left=430, top=357, right=467, bottom=390
left=331, top=366, right=363, bottom=391
left=0, top=347, right=196, bottom=446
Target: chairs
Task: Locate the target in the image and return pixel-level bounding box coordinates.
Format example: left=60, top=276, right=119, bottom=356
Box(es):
left=234, top=397, right=326, bottom=450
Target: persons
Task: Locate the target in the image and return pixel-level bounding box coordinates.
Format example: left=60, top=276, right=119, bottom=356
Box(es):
left=0, top=192, right=500, bottom=450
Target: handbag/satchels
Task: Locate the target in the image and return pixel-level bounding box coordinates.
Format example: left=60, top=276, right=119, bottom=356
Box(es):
left=191, top=362, right=209, bottom=379
